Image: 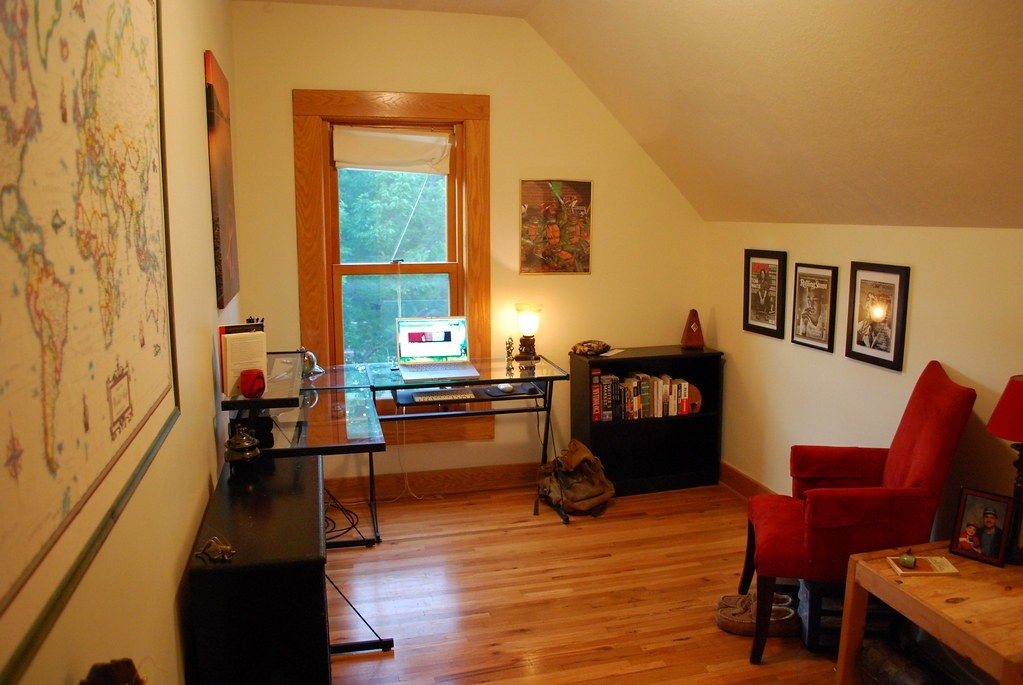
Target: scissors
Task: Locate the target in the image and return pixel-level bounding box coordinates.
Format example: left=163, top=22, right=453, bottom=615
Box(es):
left=267, top=375, right=278, bottom=383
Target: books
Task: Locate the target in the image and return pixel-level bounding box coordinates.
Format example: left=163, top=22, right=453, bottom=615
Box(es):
left=591, top=367, right=691, bottom=421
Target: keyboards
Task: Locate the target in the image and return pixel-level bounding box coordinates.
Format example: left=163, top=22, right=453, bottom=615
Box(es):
left=412, top=389, right=475, bottom=402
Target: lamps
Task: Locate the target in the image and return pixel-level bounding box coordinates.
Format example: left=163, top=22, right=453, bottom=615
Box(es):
left=985, top=375, right=1023, bottom=567
left=514, top=301, right=543, bottom=362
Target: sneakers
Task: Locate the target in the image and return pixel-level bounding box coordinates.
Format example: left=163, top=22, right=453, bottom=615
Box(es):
left=716, top=606, right=795, bottom=635
left=717, top=591, right=792, bottom=608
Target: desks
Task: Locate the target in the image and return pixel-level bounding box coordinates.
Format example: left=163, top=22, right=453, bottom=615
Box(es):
left=223, top=362, right=383, bottom=548
left=833, top=537, right=1023, bottom=685
left=366, top=354, right=569, bottom=546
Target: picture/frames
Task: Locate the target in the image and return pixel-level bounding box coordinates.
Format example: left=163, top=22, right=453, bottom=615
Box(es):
left=742, top=248, right=788, bottom=341
left=949, top=487, right=1016, bottom=568
left=791, top=262, right=839, bottom=353
left=845, top=261, right=910, bottom=372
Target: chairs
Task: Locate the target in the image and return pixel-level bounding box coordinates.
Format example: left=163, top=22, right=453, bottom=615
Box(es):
left=735, top=360, right=978, bottom=664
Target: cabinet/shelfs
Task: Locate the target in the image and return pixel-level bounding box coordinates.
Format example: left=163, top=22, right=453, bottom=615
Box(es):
left=173, top=453, right=332, bottom=685
left=569, top=342, right=726, bottom=499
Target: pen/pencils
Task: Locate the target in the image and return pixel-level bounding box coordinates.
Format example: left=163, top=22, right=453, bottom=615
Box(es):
left=276, top=367, right=294, bottom=380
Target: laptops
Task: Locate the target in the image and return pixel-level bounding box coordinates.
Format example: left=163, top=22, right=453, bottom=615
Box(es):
left=395, top=316, right=480, bottom=383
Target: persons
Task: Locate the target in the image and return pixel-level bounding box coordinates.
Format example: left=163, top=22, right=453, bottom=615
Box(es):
left=958, top=521, right=982, bottom=554
left=856, top=291, right=892, bottom=353
left=797, top=288, right=827, bottom=342
left=978, top=507, right=1002, bottom=559
left=752, top=270, right=772, bottom=321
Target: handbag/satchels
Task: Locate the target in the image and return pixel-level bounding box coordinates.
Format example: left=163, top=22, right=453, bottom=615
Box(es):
left=540, top=439, right=615, bottom=512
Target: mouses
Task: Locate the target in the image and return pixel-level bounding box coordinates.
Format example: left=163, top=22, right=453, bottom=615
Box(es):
left=498, top=384, right=514, bottom=392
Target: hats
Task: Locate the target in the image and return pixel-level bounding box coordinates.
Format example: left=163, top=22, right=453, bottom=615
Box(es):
left=983, top=507, right=998, bottom=518
left=966, top=522, right=980, bottom=528
